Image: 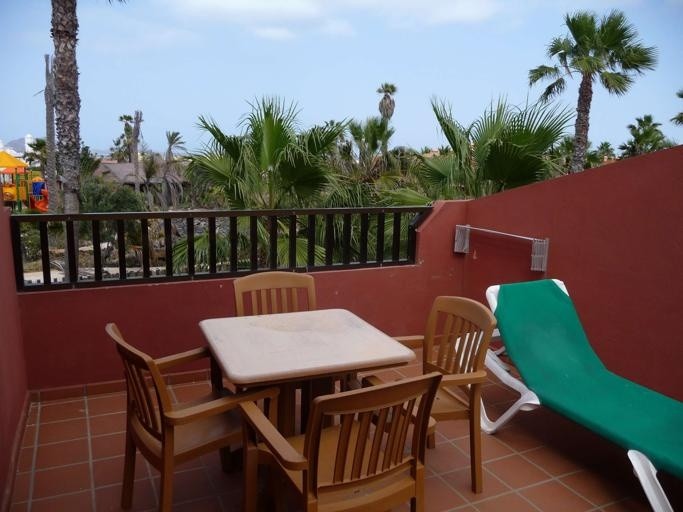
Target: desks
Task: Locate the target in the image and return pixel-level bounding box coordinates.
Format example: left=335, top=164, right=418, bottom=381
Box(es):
left=196, top=307, right=416, bottom=511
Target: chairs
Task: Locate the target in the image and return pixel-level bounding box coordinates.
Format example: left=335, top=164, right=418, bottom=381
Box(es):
left=360, top=295, right=498, bottom=494
left=454, top=276, right=683, bottom=512
left=236, top=369, right=443, bottom=512
left=105, top=322, right=280, bottom=512
left=232, top=271, right=318, bottom=438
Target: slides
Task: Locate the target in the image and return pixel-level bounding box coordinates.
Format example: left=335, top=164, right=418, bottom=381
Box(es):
left=22, top=189, right=48, bottom=212
